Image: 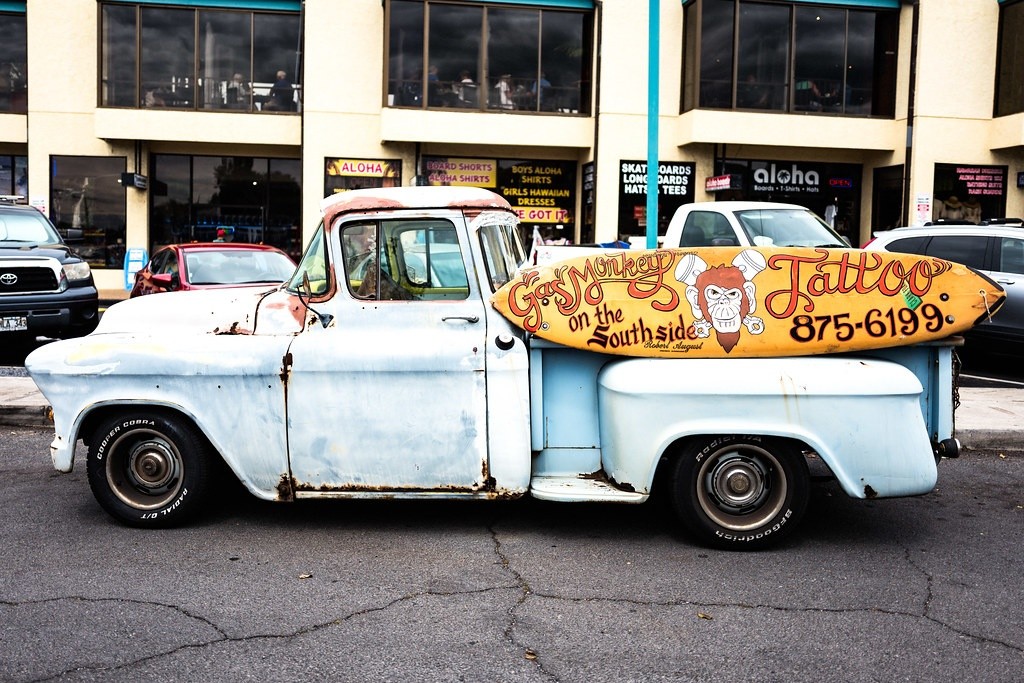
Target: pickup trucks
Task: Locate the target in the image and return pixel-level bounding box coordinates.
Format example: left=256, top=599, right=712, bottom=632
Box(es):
left=24, top=187, right=937, bottom=548
left=531, top=200, right=853, bottom=260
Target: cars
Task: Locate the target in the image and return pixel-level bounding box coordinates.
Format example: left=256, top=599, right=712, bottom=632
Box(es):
left=128, top=241, right=298, bottom=296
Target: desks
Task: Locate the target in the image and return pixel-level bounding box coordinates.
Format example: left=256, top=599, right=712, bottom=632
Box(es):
left=439, top=90, right=460, bottom=108
left=155, top=92, right=186, bottom=106
left=244, top=95, right=274, bottom=110
left=510, top=92, right=536, bottom=110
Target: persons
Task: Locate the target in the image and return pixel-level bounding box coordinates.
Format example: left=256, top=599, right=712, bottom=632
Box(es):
left=403, top=62, right=559, bottom=111
left=142, top=70, right=296, bottom=111
left=212, top=229, right=226, bottom=243
left=794, top=72, right=830, bottom=112
left=932, top=193, right=982, bottom=224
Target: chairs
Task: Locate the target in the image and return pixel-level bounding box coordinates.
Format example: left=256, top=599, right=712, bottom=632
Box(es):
left=489, top=87, right=512, bottom=111
left=457, top=85, right=478, bottom=108
left=227, top=88, right=249, bottom=111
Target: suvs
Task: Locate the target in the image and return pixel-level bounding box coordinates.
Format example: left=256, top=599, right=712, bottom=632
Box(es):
left=0, top=193, right=99, bottom=356
left=858, top=217, right=1023, bottom=387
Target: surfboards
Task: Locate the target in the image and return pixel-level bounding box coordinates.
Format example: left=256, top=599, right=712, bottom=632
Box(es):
left=487, top=247, right=1009, bottom=359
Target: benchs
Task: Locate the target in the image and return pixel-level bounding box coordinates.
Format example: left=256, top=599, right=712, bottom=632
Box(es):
left=536, top=87, right=579, bottom=111
